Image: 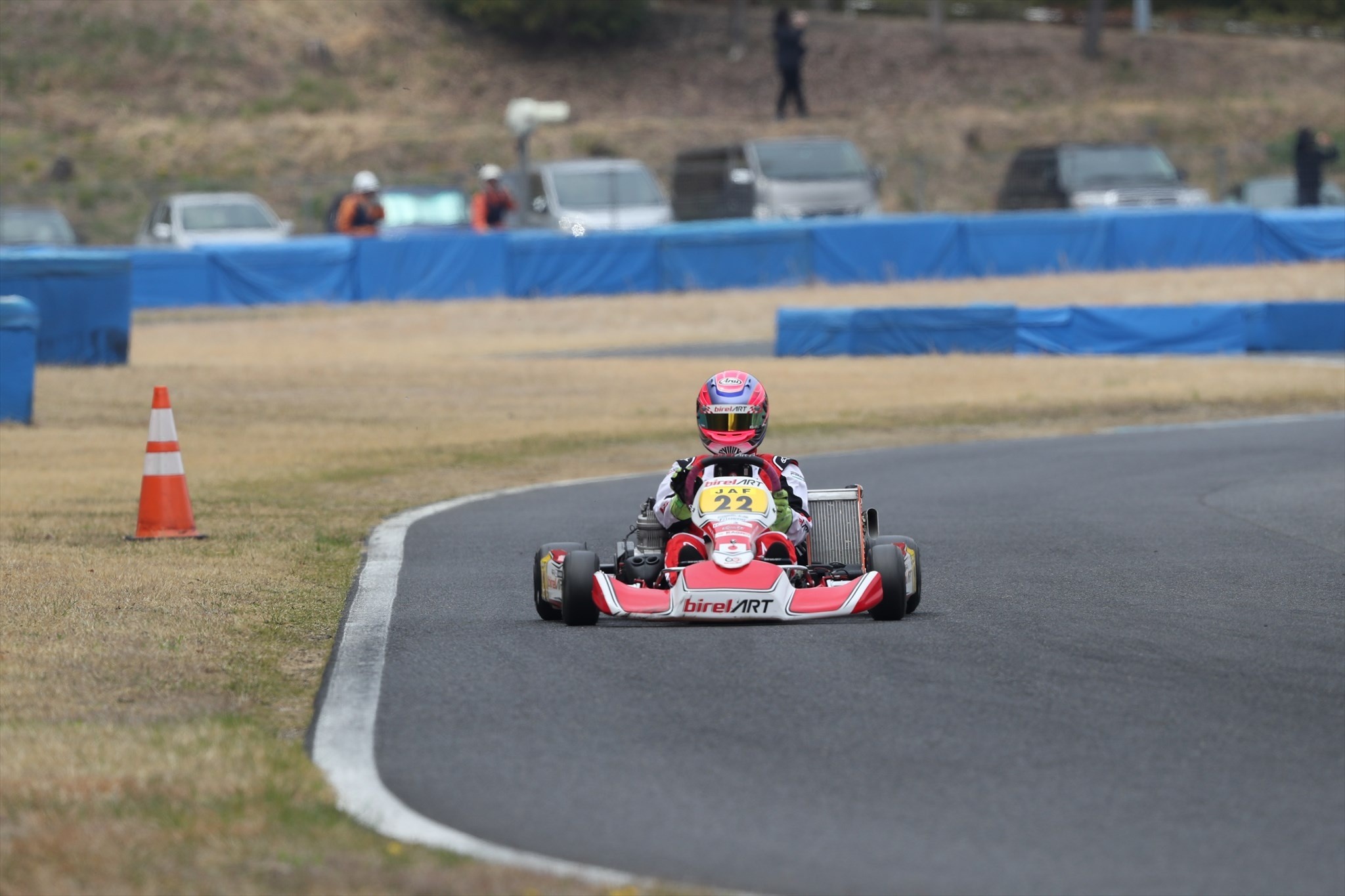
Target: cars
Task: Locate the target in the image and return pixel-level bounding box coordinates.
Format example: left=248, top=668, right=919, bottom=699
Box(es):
left=134, top=189, right=297, bottom=247
left=326, top=183, right=474, bottom=232
left=0, top=202, right=91, bottom=247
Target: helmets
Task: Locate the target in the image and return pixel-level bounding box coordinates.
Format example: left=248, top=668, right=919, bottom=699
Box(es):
left=696, top=369, right=770, bottom=458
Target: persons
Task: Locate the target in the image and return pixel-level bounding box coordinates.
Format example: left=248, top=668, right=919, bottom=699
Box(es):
left=325, top=167, right=384, bottom=234
left=1292, top=120, right=1337, bottom=211
left=656, top=370, right=812, bottom=576
left=768, top=7, right=811, bottom=121
left=468, top=163, right=512, bottom=231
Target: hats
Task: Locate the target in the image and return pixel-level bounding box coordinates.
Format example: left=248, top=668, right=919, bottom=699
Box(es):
left=478, top=164, right=502, bottom=182
left=353, top=170, right=379, bottom=195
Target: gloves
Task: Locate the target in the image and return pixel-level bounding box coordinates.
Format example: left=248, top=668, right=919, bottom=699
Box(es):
left=670, top=464, right=703, bottom=521
left=765, top=488, right=794, bottom=535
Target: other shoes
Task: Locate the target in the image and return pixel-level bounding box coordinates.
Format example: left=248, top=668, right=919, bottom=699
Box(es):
left=764, top=541, right=790, bottom=565
left=677, top=545, right=704, bottom=567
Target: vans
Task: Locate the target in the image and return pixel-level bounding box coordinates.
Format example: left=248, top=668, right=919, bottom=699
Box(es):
left=996, top=136, right=1206, bottom=213
left=676, top=131, right=884, bottom=219
left=492, top=155, right=678, bottom=230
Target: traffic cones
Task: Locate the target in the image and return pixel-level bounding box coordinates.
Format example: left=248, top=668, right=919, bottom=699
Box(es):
left=124, top=385, right=212, bottom=540
